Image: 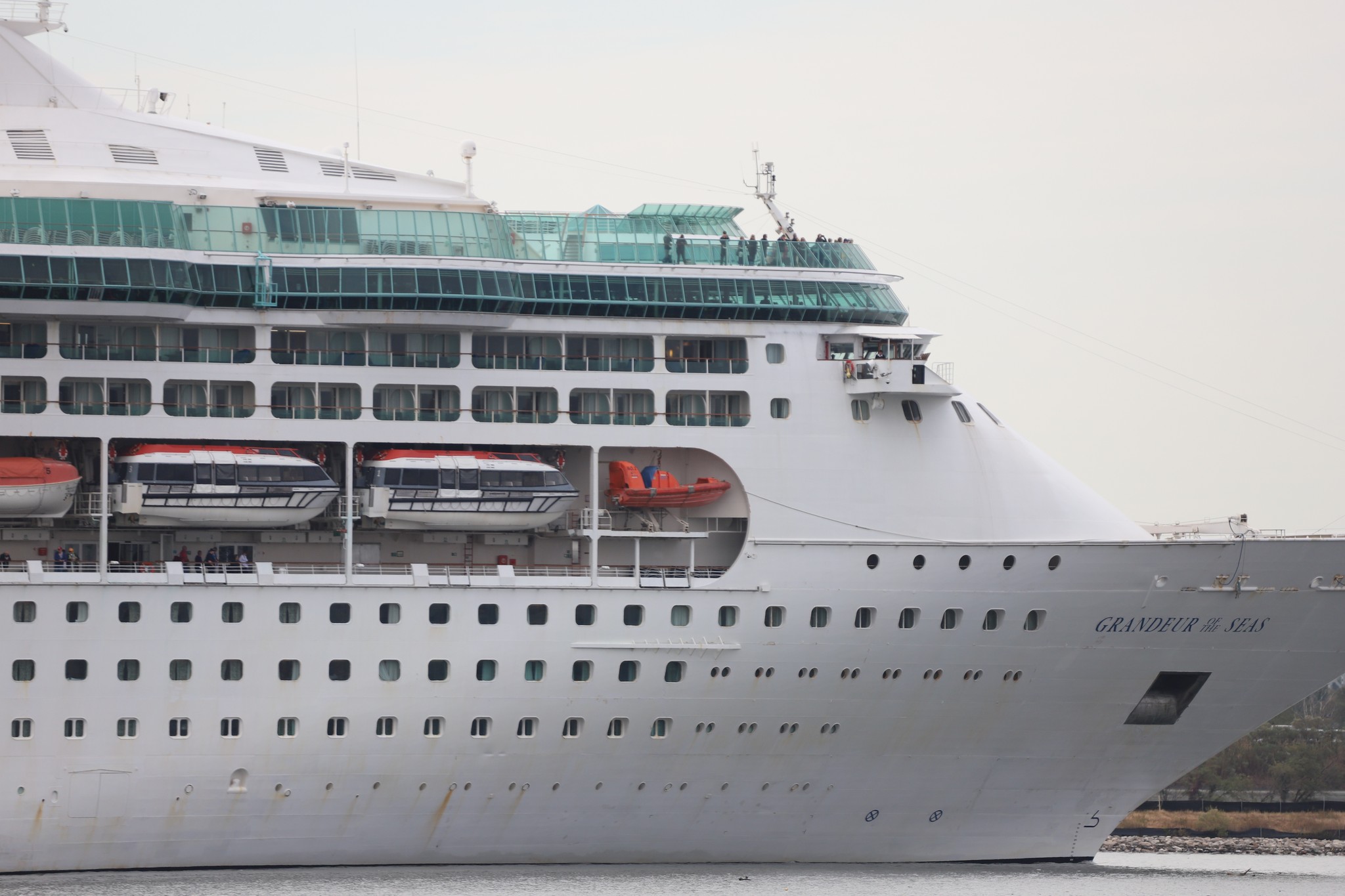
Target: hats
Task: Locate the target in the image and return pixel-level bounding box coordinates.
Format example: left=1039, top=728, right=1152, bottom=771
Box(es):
left=209, top=548, right=216, bottom=552
left=57, top=547, right=63, bottom=550
left=68, top=548, right=74, bottom=552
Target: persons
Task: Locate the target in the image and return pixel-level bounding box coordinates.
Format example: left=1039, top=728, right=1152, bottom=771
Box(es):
left=179, top=545, right=189, bottom=573
left=748, top=234, right=758, bottom=266
left=760, top=234, right=770, bottom=265
left=875, top=350, right=886, bottom=359
left=204, top=547, right=219, bottom=573
left=676, top=234, right=687, bottom=264
left=824, top=237, right=855, bottom=269
left=815, top=234, right=827, bottom=268
left=777, top=234, right=792, bottom=266
left=738, top=236, right=748, bottom=266
left=0, top=551, right=11, bottom=571
left=194, top=550, right=203, bottom=573
left=66, top=548, right=80, bottom=572
left=54, top=546, right=67, bottom=572
left=231, top=551, right=249, bottom=574
left=719, top=231, right=731, bottom=266
left=800, top=237, right=809, bottom=267
left=792, top=232, right=800, bottom=267
left=663, top=232, right=673, bottom=263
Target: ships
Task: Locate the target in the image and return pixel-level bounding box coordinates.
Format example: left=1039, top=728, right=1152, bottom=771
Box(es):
left=0, top=0, right=1345, bottom=876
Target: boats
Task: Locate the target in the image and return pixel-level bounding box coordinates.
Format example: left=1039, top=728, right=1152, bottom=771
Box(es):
left=0, top=456, right=84, bottom=516
left=360, top=450, right=579, bottom=530
left=109, top=441, right=341, bottom=528
left=603, top=460, right=733, bottom=509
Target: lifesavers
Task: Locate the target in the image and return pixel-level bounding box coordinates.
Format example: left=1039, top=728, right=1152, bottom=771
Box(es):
left=845, top=360, right=854, bottom=375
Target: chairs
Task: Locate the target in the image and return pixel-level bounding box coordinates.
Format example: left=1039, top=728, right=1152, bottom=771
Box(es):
left=480, top=481, right=522, bottom=487
left=546, top=481, right=555, bottom=486
left=242, top=476, right=294, bottom=482
